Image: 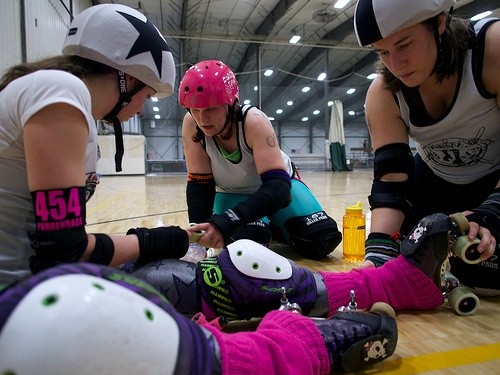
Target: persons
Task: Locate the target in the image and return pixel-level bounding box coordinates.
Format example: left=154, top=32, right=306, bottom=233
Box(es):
left=354, top=0, right=500, bottom=299
left=0, top=3, right=480, bottom=375
left=178, top=60, right=342, bottom=259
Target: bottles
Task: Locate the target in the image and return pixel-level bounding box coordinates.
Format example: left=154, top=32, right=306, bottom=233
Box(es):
left=177, top=223, right=207, bottom=265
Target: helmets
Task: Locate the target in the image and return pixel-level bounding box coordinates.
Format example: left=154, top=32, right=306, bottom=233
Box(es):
left=179, top=61, right=238, bottom=107
left=63, top=4, right=176, bottom=98
left=354, top=0, right=456, bottom=47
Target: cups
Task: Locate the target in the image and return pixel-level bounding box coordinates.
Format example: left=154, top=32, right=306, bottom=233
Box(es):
left=343, top=201, right=366, bottom=264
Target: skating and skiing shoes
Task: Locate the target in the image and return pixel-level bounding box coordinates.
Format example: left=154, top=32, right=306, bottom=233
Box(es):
left=276, top=287, right=397, bottom=375
left=400, top=214, right=483, bottom=318
